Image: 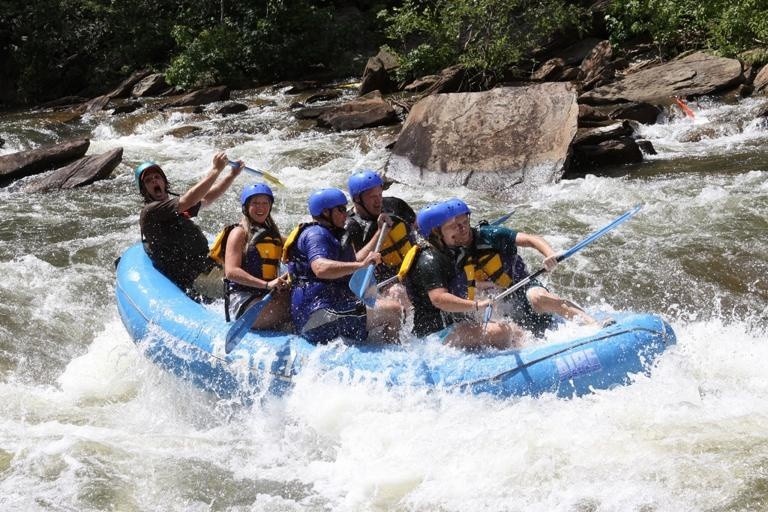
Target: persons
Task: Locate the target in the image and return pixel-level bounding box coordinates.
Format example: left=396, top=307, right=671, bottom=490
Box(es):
left=220, top=182, right=293, bottom=332
left=280, top=187, right=404, bottom=351
left=439, top=198, right=619, bottom=341
left=133, top=150, right=247, bottom=308
left=341, top=170, right=418, bottom=324
left=395, top=201, right=531, bottom=354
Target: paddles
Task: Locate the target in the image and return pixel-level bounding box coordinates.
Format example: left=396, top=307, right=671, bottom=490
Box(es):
left=481, top=293, right=496, bottom=337
left=348, top=221, right=390, bottom=308
left=226, top=161, right=288, bottom=192
left=488, top=203, right=642, bottom=302
left=485, top=208, right=515, bottom=225
left=224, top=282, right=284, bottom=354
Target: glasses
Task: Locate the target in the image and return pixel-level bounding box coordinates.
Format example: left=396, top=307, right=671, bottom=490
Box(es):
left=338, top=207, right=346, bottom=212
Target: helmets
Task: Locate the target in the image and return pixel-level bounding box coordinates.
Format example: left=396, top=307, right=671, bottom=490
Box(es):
left=240, top=183, right=274, bottom=205
left=348, top=170, right=383, bottom=198
left=135, top=163, right=168, bottom=194
left=310, top=188, right=348, bottom=216
left=447, top=198, right=471, bottom=218
left=417, top=202, right=456, bottom=236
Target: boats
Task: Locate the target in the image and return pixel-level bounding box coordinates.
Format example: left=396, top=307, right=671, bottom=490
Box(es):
left=112, top=238, right=677, bottom=402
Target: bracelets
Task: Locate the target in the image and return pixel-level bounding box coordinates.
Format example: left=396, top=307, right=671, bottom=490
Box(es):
left=475, top=302, right=478, bottom=311
left=264, top=281, right=270, bottom=291
